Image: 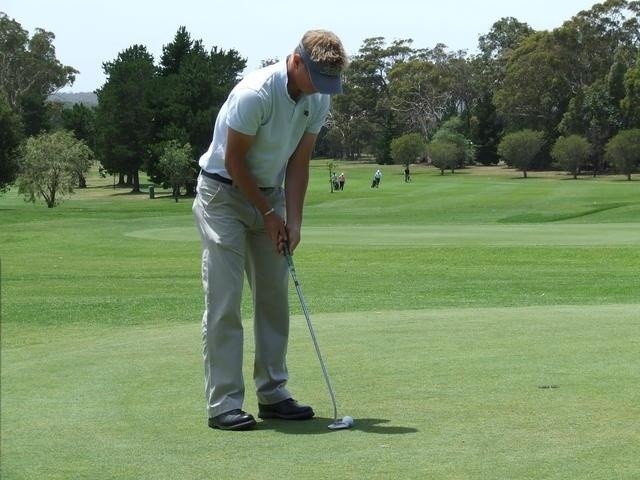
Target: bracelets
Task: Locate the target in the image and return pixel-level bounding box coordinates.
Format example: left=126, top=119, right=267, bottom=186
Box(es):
left=264, top=207, right=275, bottom=217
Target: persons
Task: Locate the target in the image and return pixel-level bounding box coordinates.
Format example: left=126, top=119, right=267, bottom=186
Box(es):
left=373, top=169, right=383, bottom=188
left=98, top=164, right=104, bottom=178
left=338, top=172, right=345, bottom=190
left=190, top=27, right=350, bottom=432
left=331, top=172, right=338, bottom=190
left=404, top=166, right=409, bottom=183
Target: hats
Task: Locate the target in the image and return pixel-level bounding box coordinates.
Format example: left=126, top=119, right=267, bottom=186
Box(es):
left=296, top=37, right=346, bottom=96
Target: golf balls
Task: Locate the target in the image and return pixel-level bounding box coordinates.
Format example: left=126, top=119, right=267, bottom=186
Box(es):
left=342, top=416, right=353, bottom=428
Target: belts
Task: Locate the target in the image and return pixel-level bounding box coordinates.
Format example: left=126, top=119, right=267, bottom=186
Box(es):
left=199, top=168, right=274, bottom=192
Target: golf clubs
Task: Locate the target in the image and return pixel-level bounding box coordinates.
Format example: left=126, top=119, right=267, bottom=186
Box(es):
left=281, top=239, right=349, bottom=431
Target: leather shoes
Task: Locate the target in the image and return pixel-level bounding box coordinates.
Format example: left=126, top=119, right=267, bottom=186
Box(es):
left=206, top=409, right=256, bottom=431
left=256, top=398, right=316, bottom=421
